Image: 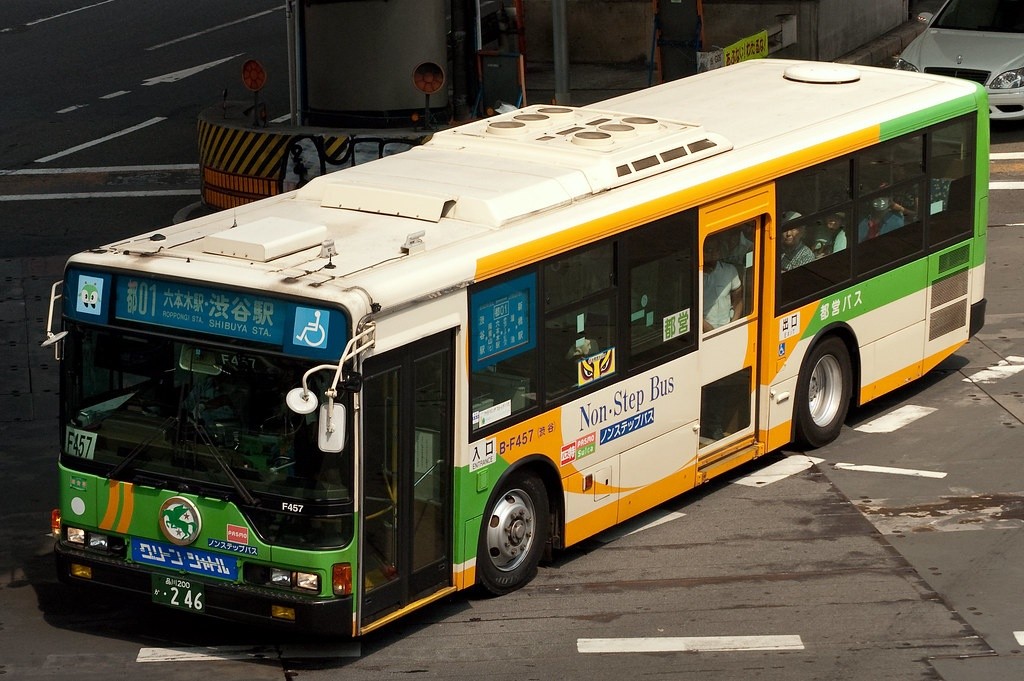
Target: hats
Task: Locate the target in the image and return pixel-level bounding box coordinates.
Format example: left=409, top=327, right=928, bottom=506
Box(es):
left=494, top=104, right=517, bottom=115
left=782, top=212, right=801, bottom=223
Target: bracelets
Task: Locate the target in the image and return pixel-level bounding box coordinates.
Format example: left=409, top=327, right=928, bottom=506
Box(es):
left=899, top=207, right=904, bottom=215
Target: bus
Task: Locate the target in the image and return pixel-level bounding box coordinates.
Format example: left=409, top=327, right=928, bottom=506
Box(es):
left=39, top=58, right=986, bottom=648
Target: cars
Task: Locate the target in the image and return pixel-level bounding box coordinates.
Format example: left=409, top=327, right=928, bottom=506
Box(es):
left=893, top=1, right=1024, bottom=122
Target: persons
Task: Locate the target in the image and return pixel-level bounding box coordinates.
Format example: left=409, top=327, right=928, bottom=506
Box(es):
left=720, top=225, right=753, bottom=265
left=891, top=164, right=918, bottom=221
left=805, top=212, right=847, bottom=258
left=549, top=324, right=600, bottom=368
left=187, top=374, right=240, bottom=421
left=858, top=197, right=903, bottom=242
left=702, top=237, right=743, bottom=440
left=781, top=211, right=815, bottom=272
